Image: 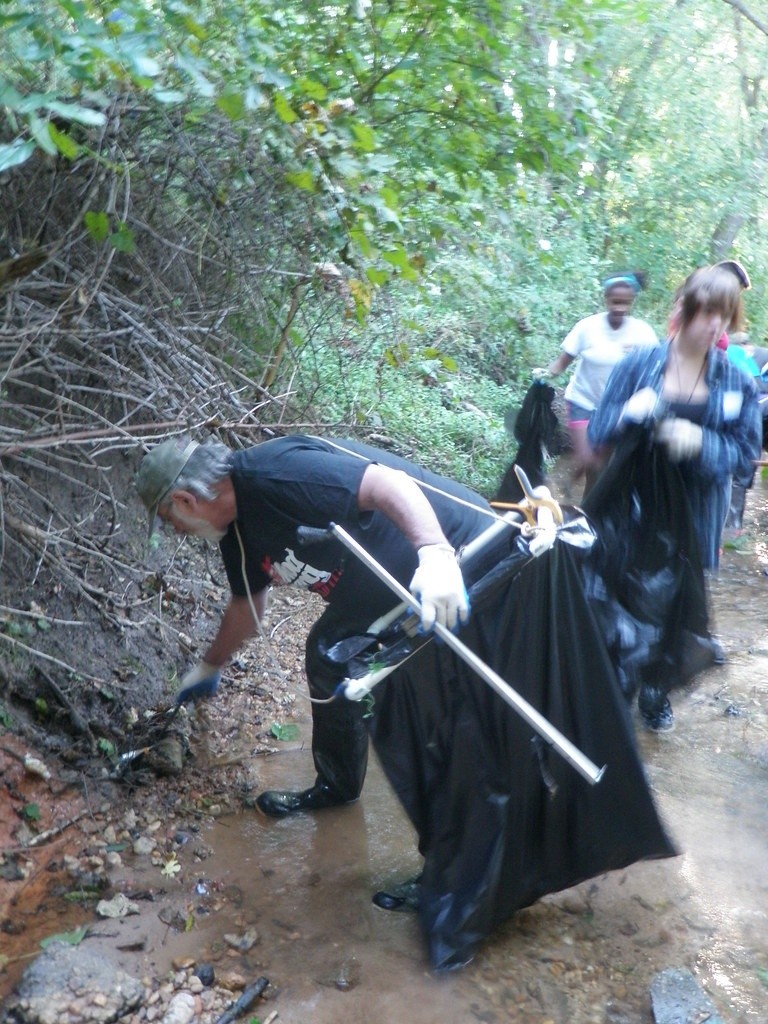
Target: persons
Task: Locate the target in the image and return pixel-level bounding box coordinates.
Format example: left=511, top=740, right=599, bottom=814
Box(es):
left=712, top=261, right=768, bottom=453
left=133, top=434, right=517, bottom=913
left=531, top=273, right=661, bottom=508
left=586, top=266, right=763, bottom=734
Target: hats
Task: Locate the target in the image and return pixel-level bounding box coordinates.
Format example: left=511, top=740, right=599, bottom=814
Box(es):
left=135, top=438, right=199, bottom=538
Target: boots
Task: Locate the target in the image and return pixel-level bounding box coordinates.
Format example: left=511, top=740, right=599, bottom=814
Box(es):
left=255, top=646, right=368, bottom=817
left=374, top=870, right=425, bottom=911
left=728, top=485, right=746, bottom=529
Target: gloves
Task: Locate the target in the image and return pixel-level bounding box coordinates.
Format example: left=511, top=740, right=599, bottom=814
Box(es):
left=625, top=386, right=660, bottom=424
left=174, top=661, right=221, bottom=701
left=663, top=418, right=703, bottom=460
left=532, top=368, right=553, bottom=382
left=409, top=542, right=471, bottom=636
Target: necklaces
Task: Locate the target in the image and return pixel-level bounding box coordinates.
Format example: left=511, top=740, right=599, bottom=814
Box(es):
left=668, top=340, right=707, bottom=418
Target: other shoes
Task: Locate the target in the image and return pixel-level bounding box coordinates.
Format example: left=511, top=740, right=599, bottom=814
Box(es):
left=640, top=687, right=676, bottom=734
